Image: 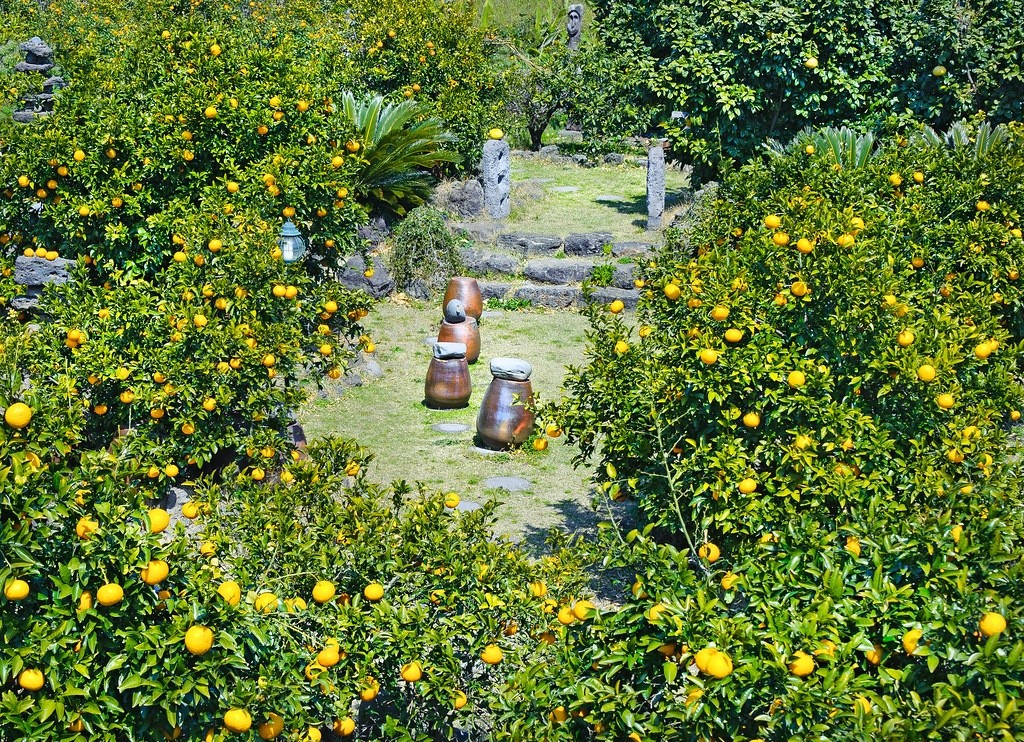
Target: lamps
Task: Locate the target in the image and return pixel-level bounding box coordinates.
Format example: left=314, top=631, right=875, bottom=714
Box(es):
left=275, top=220, right=306, bottom=266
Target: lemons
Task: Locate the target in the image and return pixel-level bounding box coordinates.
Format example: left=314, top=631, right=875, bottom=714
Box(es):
left=0, top=0, right=1024, bottom=742
